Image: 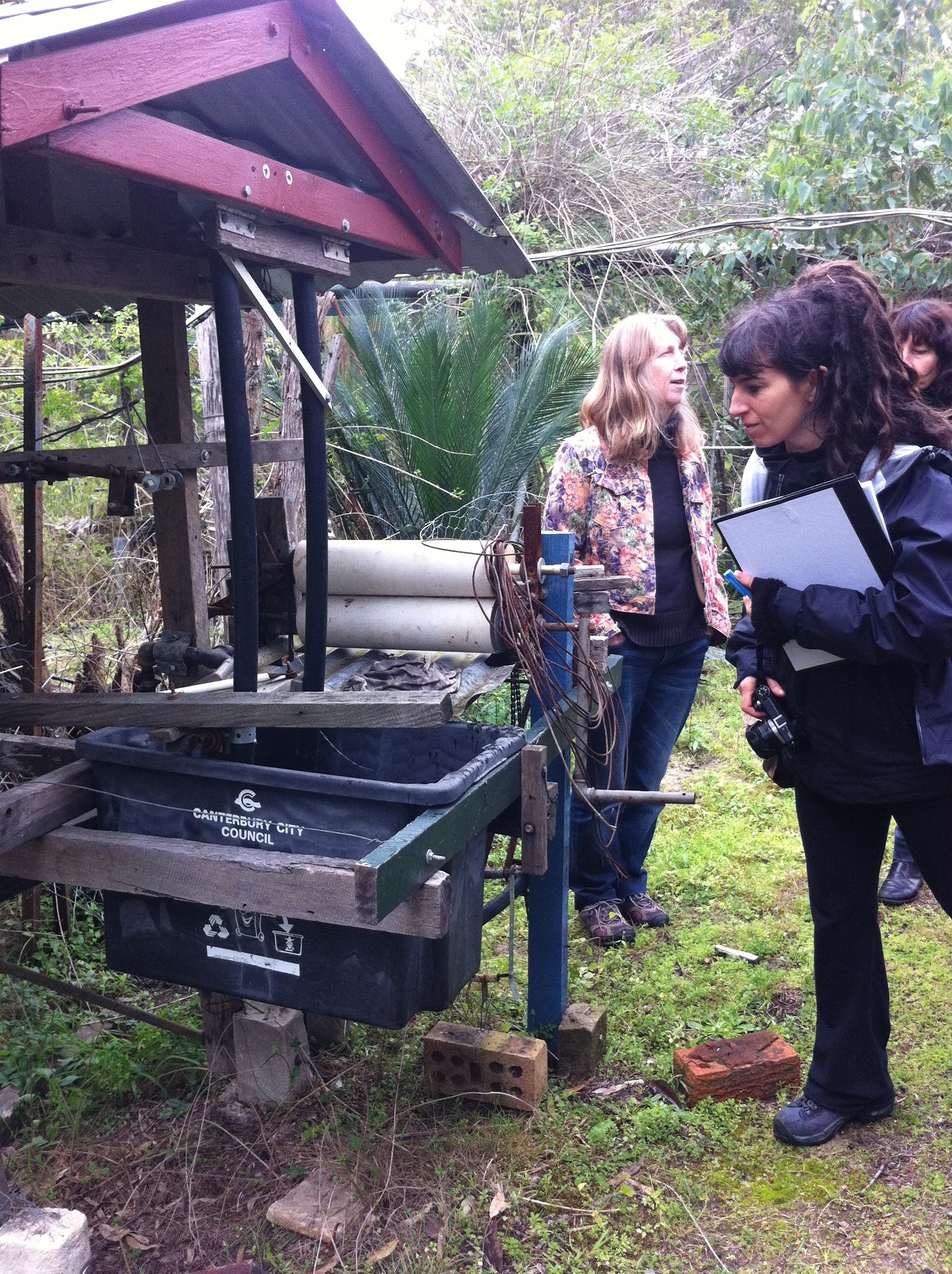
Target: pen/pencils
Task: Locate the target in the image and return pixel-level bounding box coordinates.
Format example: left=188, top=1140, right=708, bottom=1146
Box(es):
left=723, top=569, right=752, bottom=598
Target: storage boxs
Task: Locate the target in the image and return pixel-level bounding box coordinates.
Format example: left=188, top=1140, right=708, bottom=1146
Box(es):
left=75, top=722, right=526, bottom=1030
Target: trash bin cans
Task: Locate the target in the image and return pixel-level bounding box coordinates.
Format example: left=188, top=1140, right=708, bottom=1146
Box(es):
left=76, top=721, right=504, bottom=1030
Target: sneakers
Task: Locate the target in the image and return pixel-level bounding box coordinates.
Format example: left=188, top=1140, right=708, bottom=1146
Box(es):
left=616, top=893, right=670, bottom=929
left=771, top=1093, right=850, bottom=1148
left=578, top=897, right=637, bottom=948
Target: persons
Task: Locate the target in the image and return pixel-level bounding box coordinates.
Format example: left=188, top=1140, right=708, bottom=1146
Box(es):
left=720, top=294, right=952, bottom=1144
left=540, top=313, right=730, bottom=948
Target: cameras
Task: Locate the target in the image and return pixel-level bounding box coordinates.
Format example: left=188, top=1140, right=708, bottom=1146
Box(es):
left=745, top=684, right=797, bottom=760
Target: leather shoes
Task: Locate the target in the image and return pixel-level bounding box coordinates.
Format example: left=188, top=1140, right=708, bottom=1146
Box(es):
left=878, top=857, right=924, bottom=905
